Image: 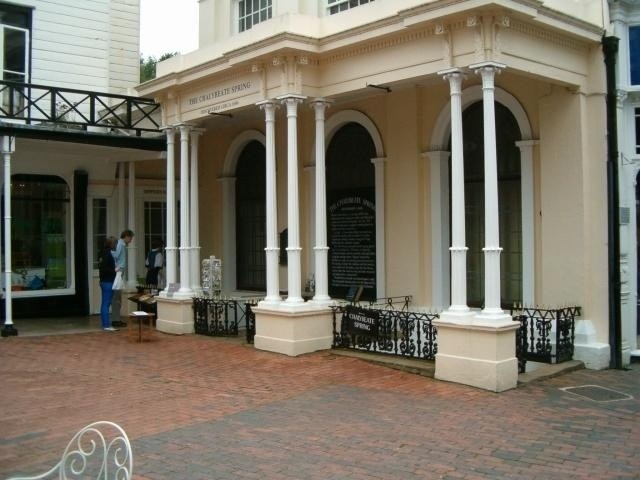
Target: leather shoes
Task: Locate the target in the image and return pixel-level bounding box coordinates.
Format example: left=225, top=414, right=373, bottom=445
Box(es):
left=113, top=321, right=126, bottom=327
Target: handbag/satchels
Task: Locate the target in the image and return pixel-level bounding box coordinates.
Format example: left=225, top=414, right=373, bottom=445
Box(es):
left=113, top=270, right=126, bottom=290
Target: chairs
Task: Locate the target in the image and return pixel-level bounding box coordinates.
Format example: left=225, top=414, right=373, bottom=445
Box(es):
left=8, top=420, right=134, bottom=479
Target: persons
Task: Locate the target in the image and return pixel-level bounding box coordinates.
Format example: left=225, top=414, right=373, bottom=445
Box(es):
left=98, top=237, right=120, bottom=331
left=145, top=240, right=166, bottom=286
left=111, top=230, right=134, bottom=327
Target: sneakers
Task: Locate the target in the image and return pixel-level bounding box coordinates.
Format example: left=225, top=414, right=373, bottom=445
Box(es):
left=104, top=327, right=119, bottom=331
